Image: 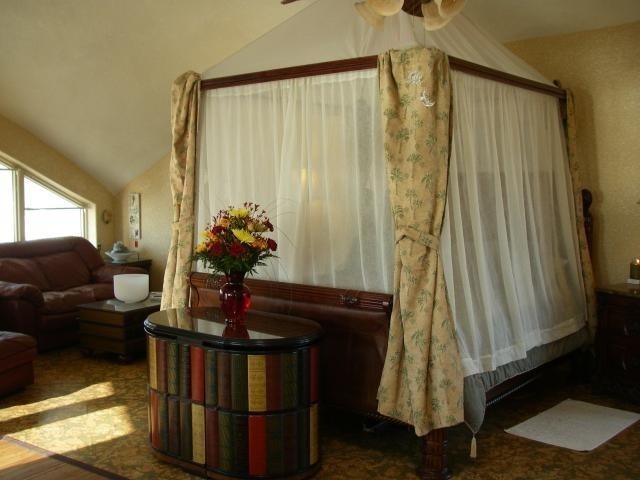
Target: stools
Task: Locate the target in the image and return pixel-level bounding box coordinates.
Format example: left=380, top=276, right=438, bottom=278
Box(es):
left=0, top=329, right=36, bottom=396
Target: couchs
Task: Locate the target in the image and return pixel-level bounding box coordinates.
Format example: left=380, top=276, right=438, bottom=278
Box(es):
left=0, top=236, right=149, bottom=355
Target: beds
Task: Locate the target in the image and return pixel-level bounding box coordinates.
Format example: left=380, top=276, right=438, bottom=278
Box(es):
left=160, top=0, right=595, bottom=479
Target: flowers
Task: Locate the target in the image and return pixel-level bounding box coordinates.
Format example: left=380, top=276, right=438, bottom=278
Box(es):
left=186, top=202, right=279, bottom=276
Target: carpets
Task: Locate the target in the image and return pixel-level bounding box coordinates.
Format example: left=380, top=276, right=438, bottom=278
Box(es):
left=504, top=398, right=640, bottom=453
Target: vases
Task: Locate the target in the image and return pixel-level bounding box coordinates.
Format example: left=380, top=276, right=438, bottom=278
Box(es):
left=216, top=273, right=252, bottom=325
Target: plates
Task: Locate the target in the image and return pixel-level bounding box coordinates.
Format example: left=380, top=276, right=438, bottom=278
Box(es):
left=102, top=252, right=137, bottom=263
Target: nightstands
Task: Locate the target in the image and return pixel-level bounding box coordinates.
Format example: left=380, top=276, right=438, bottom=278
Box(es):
left=594, top=281, right=640, bottom=405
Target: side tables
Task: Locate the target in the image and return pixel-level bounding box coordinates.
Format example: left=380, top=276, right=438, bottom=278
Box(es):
left=105, top=257, right=152, bottom=271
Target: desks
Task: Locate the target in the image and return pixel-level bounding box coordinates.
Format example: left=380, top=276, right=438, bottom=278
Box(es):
left=144, top=307, right=323, bottom=479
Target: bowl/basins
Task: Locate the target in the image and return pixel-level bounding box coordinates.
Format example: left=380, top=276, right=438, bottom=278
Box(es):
left=114, top=273, right=152, bottom=302
